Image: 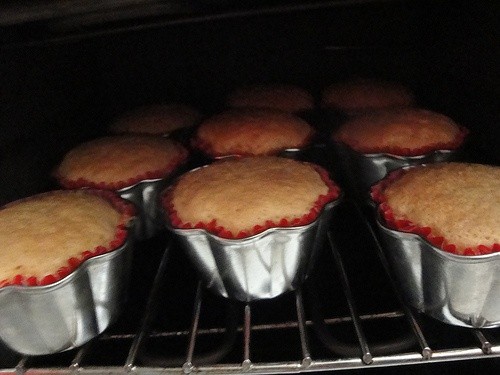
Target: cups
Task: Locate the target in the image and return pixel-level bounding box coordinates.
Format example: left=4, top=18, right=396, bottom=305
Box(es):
left=369, top=184, right=500, bottom=330
left=117, top=170, right=175, bottom=240
left=341, top=141, right=454, bottom=209
left=1, top=206, right=134, bottom=355
left=161, top=194, right=336, bottom=300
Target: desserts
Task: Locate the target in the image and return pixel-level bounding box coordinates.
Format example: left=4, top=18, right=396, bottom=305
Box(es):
left=0, top=75, right=500, bottom=357
